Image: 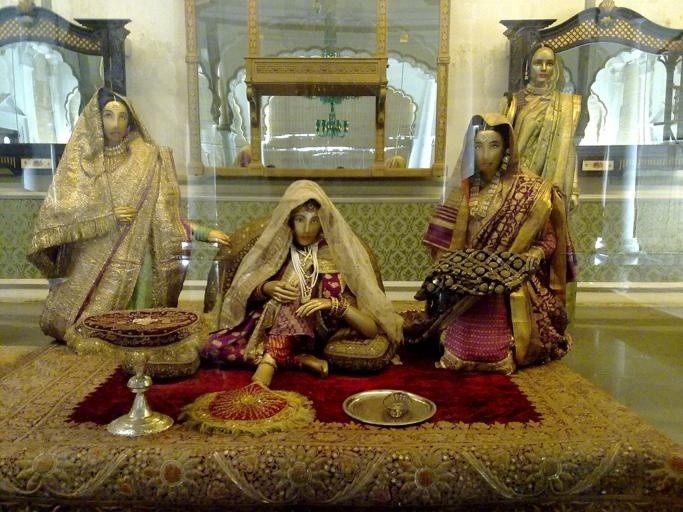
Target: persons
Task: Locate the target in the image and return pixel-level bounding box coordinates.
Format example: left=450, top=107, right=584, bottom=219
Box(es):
left=493, top=43, right=582, bottom=209
left=198, top=179, right=403, bottom=387
left=25, top=87, right=235, bottom=340
left=410, top=110, right=571, bottom=377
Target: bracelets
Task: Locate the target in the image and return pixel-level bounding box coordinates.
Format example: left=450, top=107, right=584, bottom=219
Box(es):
left=195, top=226, right=211, bottom=242
left=253, top=281, right=270, bottom=301
left=327, top=296, right=350, bottom=319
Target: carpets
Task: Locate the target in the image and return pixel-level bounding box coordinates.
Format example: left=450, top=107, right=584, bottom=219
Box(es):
left=1, top=302, right=683, bottom=512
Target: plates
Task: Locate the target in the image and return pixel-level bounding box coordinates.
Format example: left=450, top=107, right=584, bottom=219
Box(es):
left=341, top=388, right=438, bottom=427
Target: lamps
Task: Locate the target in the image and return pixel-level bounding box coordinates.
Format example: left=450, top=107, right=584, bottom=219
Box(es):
left=316, top=1, right=351, bottom=137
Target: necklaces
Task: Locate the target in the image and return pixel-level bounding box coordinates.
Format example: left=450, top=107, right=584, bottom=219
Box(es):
left=289, top=241, right=318, bottom=305
left=102, top=140, right=128, bottom=157
left=468, top=171, right=500, bottom=218
left=526, top=83, right=548, bottom=95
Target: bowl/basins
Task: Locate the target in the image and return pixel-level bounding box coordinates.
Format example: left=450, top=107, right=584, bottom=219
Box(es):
left=381, top=391, right=413, bottom=418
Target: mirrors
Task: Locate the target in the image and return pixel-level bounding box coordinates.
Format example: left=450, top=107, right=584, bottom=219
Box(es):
left=499, top=0, right=682, bottom=178
left=0, top=1, right=132, bottom=174
left=186, top=0, right=448, bottom=180
